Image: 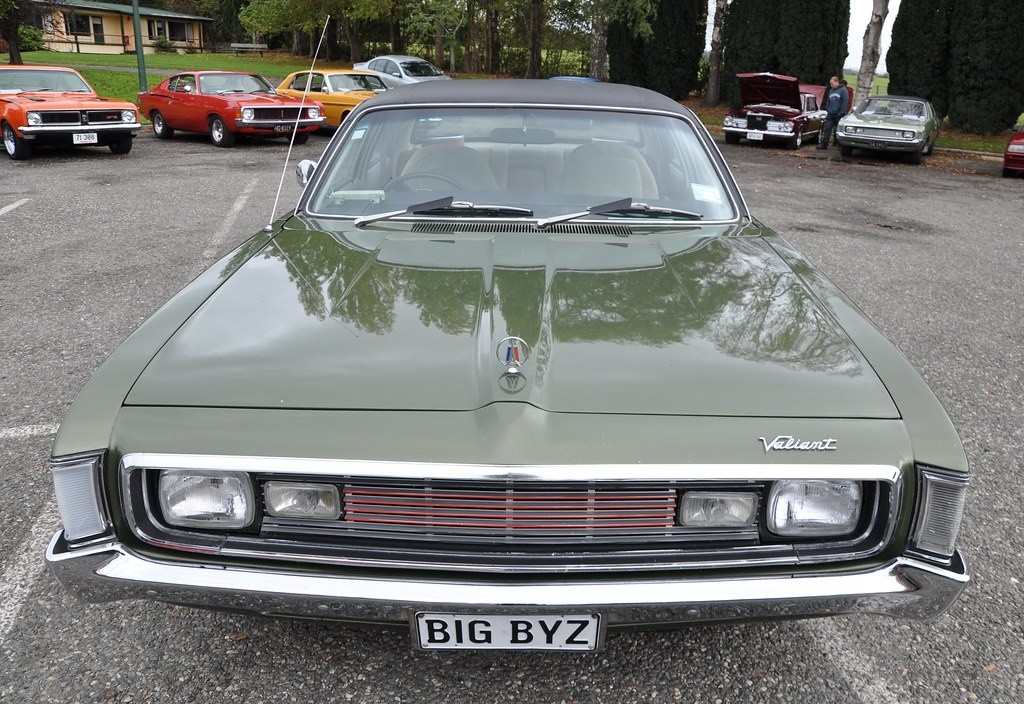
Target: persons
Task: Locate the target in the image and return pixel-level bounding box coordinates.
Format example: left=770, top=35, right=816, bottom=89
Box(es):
left=817, top=76, right=853, bottom=150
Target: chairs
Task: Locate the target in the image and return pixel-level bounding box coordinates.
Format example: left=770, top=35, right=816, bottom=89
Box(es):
left=401, top=144, right=500, bottom=195
left=562, top=140, right=661, bottom=200
left=38, top=77, right=60, bottom=91
left=409, top=64, right=424, bottom=76
left=185, top=81, right=245, bottom=94
left=872, top=106, right=887, bottom=113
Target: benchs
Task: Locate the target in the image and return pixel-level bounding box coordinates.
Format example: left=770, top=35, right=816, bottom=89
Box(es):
left=398, top=143, right=581, bottom=195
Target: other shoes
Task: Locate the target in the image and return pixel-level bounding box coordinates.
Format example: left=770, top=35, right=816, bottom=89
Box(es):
left=817, top=143, right=828, bottom=150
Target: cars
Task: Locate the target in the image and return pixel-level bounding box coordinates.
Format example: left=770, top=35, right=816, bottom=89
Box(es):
left=275, top=69, right=392, bottom=130
left=352, top=53, right=452, bottom=88
left=1001, top=122, right=1024, bottom=177
left=44, top=77, right=972, bottom=655
left=136, top=71, right=327, bottom=147
left=835, top=95, right=943, bottom=164
left=723, top=73, right=829, bottom=149
left=0, top=63, right=142, bottom=160
